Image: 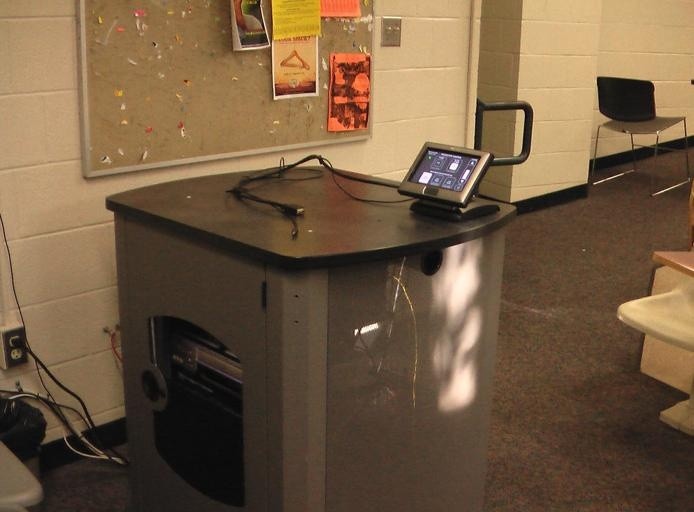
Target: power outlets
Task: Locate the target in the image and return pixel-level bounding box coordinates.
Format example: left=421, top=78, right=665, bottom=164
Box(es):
left=0, top=323, right=28, bottom=371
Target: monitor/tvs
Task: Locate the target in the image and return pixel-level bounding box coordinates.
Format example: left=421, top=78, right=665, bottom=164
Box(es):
left=397, top=140, right=500, bottom=221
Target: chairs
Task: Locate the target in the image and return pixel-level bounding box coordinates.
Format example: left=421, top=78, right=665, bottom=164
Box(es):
left=590, top=77, right=691, bottom=196
